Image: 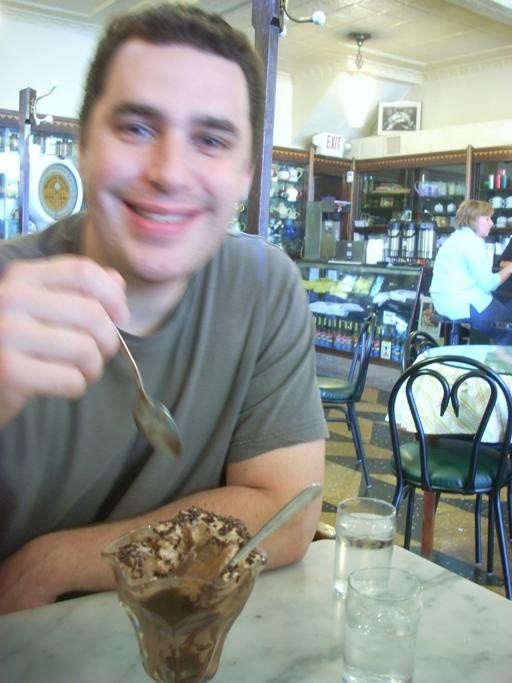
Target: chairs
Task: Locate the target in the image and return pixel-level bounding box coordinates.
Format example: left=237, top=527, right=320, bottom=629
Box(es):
left=402, top=331, right=440, bottom=373
left=314, top=312, right=377, bottom=488
left=388, top=355, right=512, bottom=599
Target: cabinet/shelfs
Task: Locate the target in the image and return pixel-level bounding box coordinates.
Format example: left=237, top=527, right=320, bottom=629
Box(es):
left=0, top=108, right=312, bottom=258
left=353, top=145, right=512, bottom=258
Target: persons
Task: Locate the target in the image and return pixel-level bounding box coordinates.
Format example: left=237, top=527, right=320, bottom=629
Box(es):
left=1, top=3, right=332, bottom=616
left=498, top=237, right=512, bottom=268
left=430, top=199, right=512, bottom=345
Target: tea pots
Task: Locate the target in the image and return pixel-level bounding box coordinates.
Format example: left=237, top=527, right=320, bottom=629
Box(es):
left=227, top=201, right=248, bottom=233
left=269, top=164, right=302, bottom=220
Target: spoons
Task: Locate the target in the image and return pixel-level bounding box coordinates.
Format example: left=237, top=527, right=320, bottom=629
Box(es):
left=115, top=325, right=184, bottom=466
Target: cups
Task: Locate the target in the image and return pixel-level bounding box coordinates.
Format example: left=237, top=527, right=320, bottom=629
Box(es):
left=489, top=194, right=512, bottom=207
left=334, top=561, right=424, bottom=681
left=329, top=496, right=398, bottom=605
left=412, top=178, right=466, bottom=198
left=434, top=200, right=456, bottom=213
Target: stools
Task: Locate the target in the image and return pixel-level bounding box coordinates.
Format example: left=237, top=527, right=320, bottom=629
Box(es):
left=429, top=316, right=468, bottom=345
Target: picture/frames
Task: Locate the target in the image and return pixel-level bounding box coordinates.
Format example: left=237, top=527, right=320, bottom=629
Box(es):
left=377, top=100, right=422, bottom=135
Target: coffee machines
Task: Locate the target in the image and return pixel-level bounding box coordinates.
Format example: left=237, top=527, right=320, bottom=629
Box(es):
left=303, top=200, right=350, bottom=261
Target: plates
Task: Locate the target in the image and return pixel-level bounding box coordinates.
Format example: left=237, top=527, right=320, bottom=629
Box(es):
left=37, top=162, right=78, bottom=220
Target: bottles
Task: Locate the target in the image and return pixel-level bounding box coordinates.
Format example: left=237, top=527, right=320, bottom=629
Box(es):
left=481, top=173, right=508, bottom=190
left=311, top=316, right=408, bottom=363
